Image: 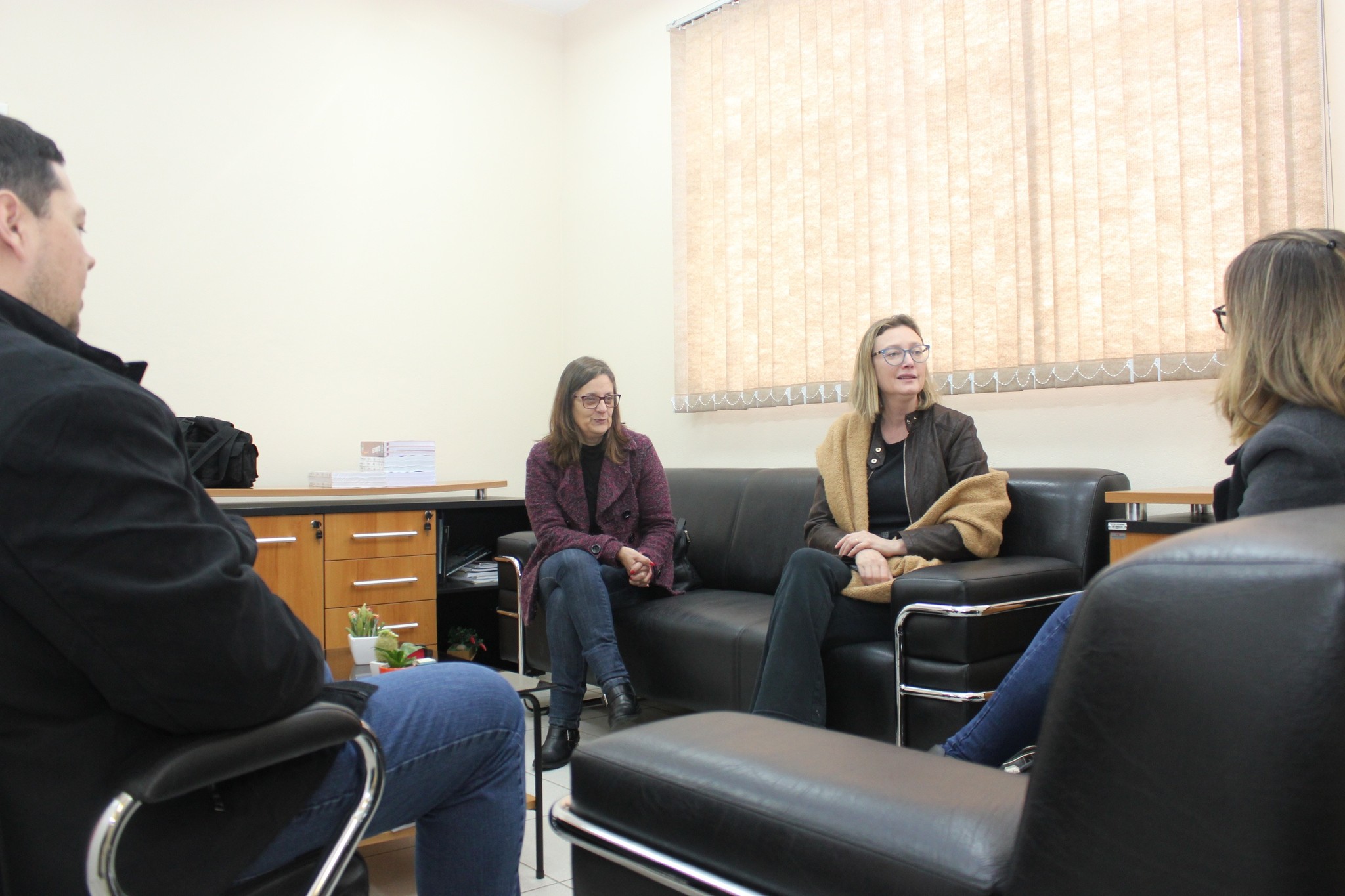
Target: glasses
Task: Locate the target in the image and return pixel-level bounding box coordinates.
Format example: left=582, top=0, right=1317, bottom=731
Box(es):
left=871, top=344, right=930, bottom=366
left=1213, top=304, right=1226, bottom=333
left=574, top=394, right=621, bottom=409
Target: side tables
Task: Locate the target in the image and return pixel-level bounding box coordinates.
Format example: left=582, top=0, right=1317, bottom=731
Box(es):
left=1105, top=487, right=1215, bottom=567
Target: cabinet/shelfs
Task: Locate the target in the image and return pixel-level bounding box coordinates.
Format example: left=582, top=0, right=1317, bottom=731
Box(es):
left=437, top=572, right=508, bottom=663
left=326, top=510, right=437, bottom=652
left=243, top=514, right=324, bottom=650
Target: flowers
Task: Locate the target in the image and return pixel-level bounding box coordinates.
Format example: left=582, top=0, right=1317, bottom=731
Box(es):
left=346, top=606, right=385, bottom=637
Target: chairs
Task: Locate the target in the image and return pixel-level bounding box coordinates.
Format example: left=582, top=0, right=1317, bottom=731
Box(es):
left=85, top=699, right=389, bottom=896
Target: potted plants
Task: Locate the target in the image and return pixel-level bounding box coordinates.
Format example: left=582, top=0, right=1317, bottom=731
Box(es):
left=372, top=641, right=427, bottom=673
left=370, top=630, right=400, bottom=677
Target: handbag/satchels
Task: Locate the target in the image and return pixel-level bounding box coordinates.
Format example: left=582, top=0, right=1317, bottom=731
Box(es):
left=178, top=417, right=258, bottom=488
left=653, top=518, right=702, bottom=601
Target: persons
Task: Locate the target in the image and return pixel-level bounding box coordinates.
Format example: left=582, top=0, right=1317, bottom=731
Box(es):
left=520, top=357, right=678, bottom=769
left=933, top=230, right=1345, bottom=777
left=749, top=315, right=1009, bottom=728
left=0, top=114, right=525, bottom=895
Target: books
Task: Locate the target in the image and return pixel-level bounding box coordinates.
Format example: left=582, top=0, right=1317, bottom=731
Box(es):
left=310, top=441, right=436, bottom=487
left=445, top=546, right=500, bottom=584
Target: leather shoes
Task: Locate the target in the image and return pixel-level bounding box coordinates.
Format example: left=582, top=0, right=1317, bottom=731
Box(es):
left=602, top=684, right=641, bottom=730
left=532, top=725, right=580, bottom=769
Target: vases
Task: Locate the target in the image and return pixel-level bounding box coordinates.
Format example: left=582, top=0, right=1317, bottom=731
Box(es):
left=348, top=633, right=380, bottom=665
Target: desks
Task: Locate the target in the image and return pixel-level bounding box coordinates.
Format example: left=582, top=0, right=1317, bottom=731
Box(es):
left=210, top=478, right=529, bottom=668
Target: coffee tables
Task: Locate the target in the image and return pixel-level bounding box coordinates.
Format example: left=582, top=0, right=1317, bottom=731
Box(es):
left=324, top=647, right=557, bottom=878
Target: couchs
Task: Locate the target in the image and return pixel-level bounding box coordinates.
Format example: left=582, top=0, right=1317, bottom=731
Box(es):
left=496, top=464, right=1130, bottom=751
left=548, top=498, right=1345, bottom=896
